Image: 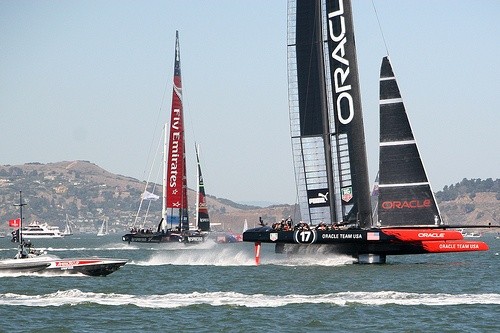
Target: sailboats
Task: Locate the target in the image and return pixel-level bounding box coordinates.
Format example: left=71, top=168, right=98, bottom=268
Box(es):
left=60, top=214, right=75, bottom=237
left=121, top=28, right=245, bottom=243
left=244, top=0, right=490, bottom=267
left=96, top=216, right=111, bottom=237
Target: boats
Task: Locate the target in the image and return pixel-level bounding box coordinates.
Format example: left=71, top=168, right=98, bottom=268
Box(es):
left=0, top=189, right=129, bottom=278
left=21, top=220, right=64, bottom=240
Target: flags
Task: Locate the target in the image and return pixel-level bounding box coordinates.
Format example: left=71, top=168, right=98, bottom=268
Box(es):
left=8, top=218, right=21, bottom=229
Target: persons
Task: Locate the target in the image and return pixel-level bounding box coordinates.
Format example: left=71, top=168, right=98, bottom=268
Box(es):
left=333, top=223, right=337, bottom=230
left=266, top=215, right=293, bottom=231
left=316, top=221, right=326, bottom=231
